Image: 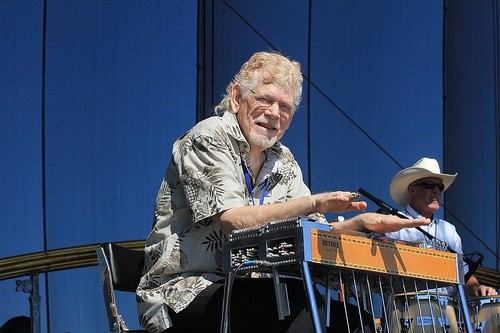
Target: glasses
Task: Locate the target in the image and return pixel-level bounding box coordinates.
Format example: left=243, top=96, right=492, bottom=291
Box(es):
left=239, top=82, right=296, bottom=115
left=410, top=181, right=446, bottom=192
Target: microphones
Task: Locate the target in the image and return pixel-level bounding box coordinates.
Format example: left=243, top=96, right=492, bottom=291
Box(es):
left=357, top=187, right=398, bottom=217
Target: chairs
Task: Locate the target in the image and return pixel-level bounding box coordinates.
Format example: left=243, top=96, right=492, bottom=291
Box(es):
left=97, top=241, right=149, bottom=333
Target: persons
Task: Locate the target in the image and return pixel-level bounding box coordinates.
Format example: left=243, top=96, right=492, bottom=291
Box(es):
left=368, top=157, right=500, bottom=333
left=136, top=51, right=431, bottom=333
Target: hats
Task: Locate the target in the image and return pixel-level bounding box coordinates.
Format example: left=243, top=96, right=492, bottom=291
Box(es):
left=389, top=157, right=458, bottom=207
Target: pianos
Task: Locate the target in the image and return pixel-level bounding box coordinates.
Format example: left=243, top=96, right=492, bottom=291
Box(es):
left=221, top=216, right=475, bottom=333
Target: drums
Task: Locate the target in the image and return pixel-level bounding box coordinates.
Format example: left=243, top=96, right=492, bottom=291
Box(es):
left=461, top=295, right=500, bottom=333
left=381, top=290, right=461, bottom=333
